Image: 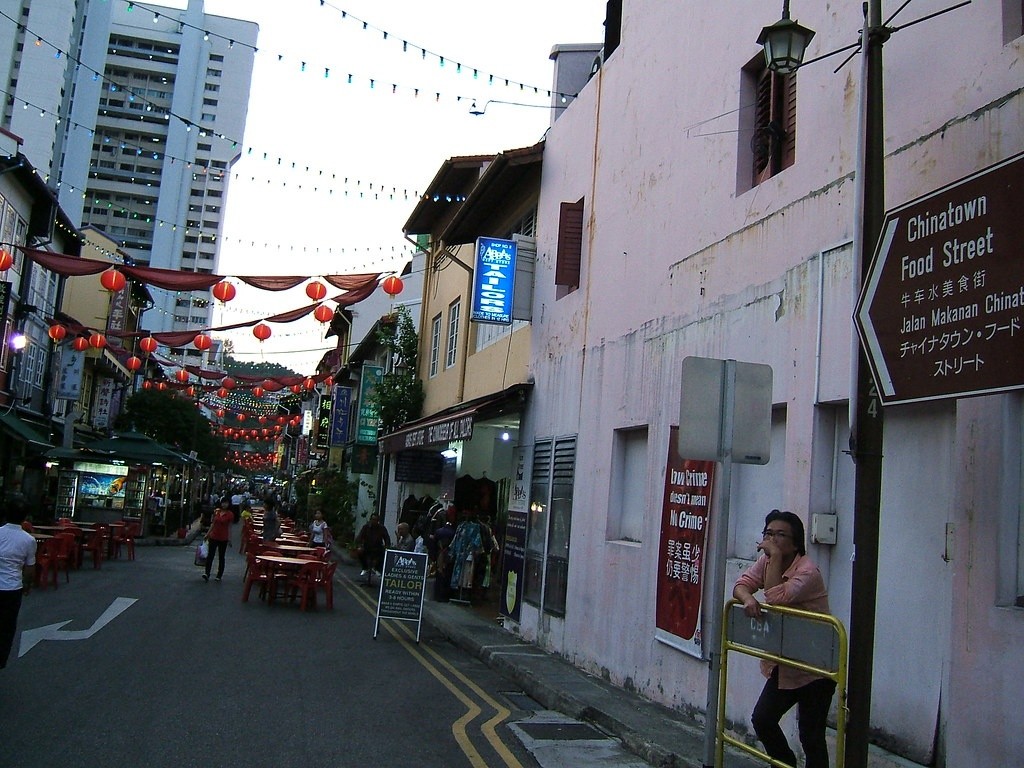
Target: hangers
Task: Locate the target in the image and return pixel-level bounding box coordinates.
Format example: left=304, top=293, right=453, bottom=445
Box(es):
left=462, top=514, right=482, bottom=530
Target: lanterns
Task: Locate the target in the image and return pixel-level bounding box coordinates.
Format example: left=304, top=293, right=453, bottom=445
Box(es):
left=0, top=249, right=402, bottom=371
left=143, top=370, right=331, bottom=442
left=225, top=452, right=272, bottom=471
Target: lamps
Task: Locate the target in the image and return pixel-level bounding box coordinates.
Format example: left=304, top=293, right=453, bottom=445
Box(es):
left=52, top=412, right=62, bottom=417
left=441, top=449, right=458, bottom=459
left=15, top=397, right=32, bottom=405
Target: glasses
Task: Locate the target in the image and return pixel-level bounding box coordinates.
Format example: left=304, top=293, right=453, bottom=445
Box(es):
left=762, top=530, right=792, bottom=539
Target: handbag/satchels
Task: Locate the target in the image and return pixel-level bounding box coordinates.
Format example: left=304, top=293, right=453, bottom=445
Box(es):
left=321, top=523, right=336, bottom=552
left=194, top=539, right=209, bottom=566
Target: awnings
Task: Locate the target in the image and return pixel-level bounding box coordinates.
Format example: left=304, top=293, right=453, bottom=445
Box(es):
left=378, top=396, right=502, bottom=454
left=54, top=425, right=106, bottom=444
left=0, top=411, right=55, bottom=448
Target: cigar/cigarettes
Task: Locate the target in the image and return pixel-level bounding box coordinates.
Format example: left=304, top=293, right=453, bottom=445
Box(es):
left=756, top=542, right=760, bottom=545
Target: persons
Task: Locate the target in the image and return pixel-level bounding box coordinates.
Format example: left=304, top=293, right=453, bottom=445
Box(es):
left=733, top=509, right=837, bottom=768
left=202, top=497, right=233, bottom=581
left=0, top=494, right=37, bottom=670
left=400, top=493, right=455, bottom=578
left=213, top=489, right=295, bottom=524
left=308, top=509, right=455, bottom=603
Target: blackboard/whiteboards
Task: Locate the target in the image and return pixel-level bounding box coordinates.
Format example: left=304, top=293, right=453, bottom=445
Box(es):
left=378, top=550, right=429, bottom=620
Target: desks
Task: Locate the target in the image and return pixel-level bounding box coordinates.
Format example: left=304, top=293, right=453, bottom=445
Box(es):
left=29, top=533, right=54, bottom=541
left=256, top=555, right=329, bottom=607
left=32, top=526, right=97, bottom=570
left=71, top=521, right=123, bottom=559
left=251, top=507, right=316, bottom=552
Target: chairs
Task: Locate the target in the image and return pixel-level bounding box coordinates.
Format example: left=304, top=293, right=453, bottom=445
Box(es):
left=22, top=518, right=140, bottom=591
left=244, top=517, right=337, bottom=611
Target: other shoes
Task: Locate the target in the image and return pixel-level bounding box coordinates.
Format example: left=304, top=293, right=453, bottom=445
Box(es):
left=215, top=577, right=221, bottom=581
left=360, top=569, right=370, bottom=576
left=375, top=570, right=381, bottom=576
left=202, top=574, right=209, bottom=581
